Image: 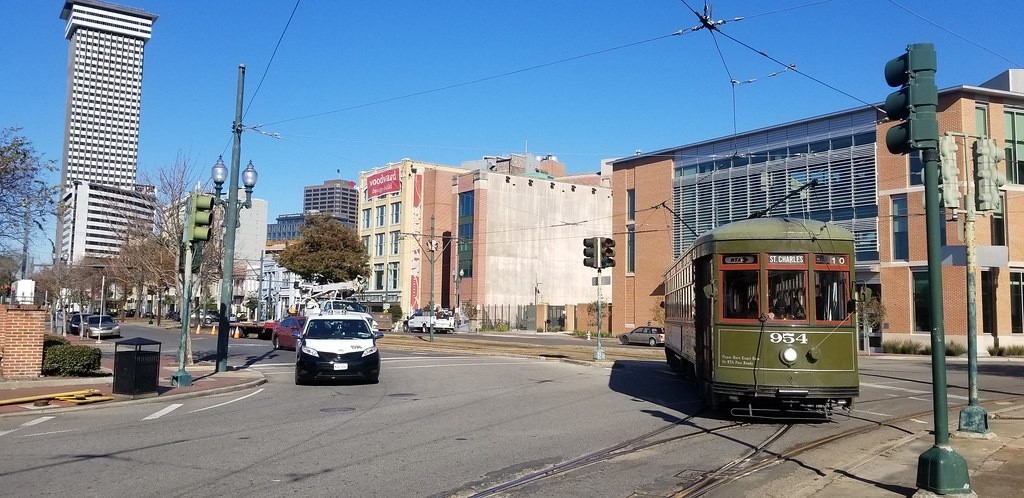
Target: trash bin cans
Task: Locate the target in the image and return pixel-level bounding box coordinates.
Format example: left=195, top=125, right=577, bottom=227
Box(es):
left=469, top=319, right=477, bottom=331
left=112, top=337, right=162, bottom=394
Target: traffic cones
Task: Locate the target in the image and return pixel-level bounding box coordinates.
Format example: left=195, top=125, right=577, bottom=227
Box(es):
left=195, top=324, right=202, bottom=335
left=209, top=325, right=217, bottom=335
left=233, top=326, right=240, bottom=340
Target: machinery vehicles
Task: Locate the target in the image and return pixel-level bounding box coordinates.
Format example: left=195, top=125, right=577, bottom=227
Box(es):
left=232, top=274, right=380, bottom=340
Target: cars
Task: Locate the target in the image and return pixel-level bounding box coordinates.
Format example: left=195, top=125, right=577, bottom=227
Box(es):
left=290, top=309, right=382, bottom=386
left=165, top=310, right=240, bottom=325
left=271, top=315, right=332, bottom=351
left=67, top=306, right=153, bottom=339
left=618, top=325, right=666, bottom=348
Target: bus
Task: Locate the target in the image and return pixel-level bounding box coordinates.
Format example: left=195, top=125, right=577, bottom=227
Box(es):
left=661, top=177, right=873, bottom=423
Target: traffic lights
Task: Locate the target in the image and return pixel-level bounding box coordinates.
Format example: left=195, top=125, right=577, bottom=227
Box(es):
left=599, top=237, right=616, bottom=268
left=883, top=41, right=940, bottom=155
left=971, top=139, right=1007, bottom=211
left=186, top=193, right=215, bottom=247
left=918, top=136, right=962, bottom=209
left=582, top=237, right=598, bottom=268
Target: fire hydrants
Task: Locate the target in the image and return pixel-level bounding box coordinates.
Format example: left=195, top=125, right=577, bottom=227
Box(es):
left=586, top=331, right=592, bottom=341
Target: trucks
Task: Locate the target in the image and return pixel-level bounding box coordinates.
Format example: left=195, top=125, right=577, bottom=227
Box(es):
left=402, top=309, right=455, bottom=333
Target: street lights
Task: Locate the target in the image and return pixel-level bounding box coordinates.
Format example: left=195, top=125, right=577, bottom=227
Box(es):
left=207, top=60, right=259, bottom=374
left=451, top=268, right=466, bottom=334
left=50, top=250, right=70, bottom=330
